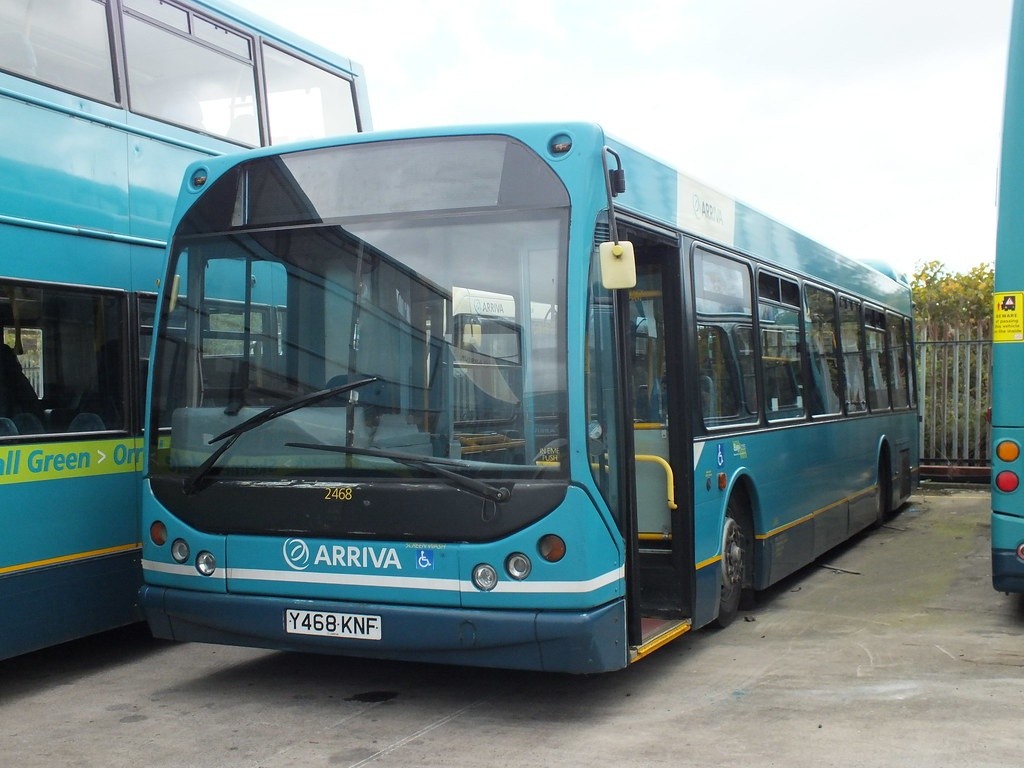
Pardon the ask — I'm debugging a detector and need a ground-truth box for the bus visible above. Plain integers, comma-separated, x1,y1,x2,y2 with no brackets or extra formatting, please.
991,0,1024,593
137,120,924,673
0,0,375,661
